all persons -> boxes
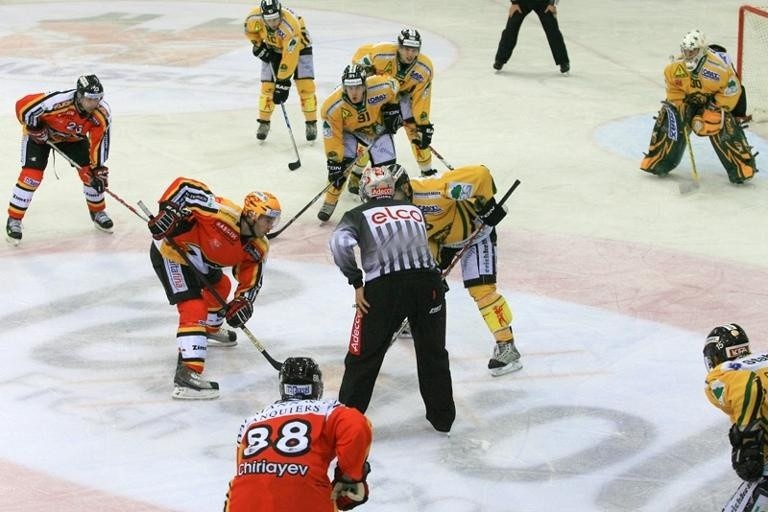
[222,353,373,512]
[243,1,318,140]
[640,30,758,184]
[348,26,435,196]
[4,74,112,240]
[149,176,279,390]
[702,322,768,512]
[376,162,521,370]
[494,0,570,73]
[315,64,398,223]
[330,165,456,432]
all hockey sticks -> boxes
[268,61,301,171]
[266,128,390,239]
[136,200,283,370]
[679,111,700,193]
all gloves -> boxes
[27,124,48,144]
[382,104,433,149]
[476,197,506,226]
[89,166,108,193]
[273,80,290,103]
[330,461,371,511]
[253,43,272,62]
[327,164,345,188]
[148,201,181,240]
[729,423,764,481]
[218,296,253,328]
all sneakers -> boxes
[7,218,22,239]
[494,61,503,69]
[395,320,410,334]
[306,120,317,140]
[257,119,270,139]
[208,328,236,342]
[91,211,113,228]
[561,62,569,72]
[489,340,520,368]
[174,368,219,390]
[348,171,362,194]
[318,202,335,221]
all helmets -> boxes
[681,29,702,50]
[703,324,749,372]
[342,64,367,86]
[243,191,281,222]
[359,164,409,200]
[279,358,323,401]
[77,75,103,99]
[259,0,281,20]
[398,28,421,48]
